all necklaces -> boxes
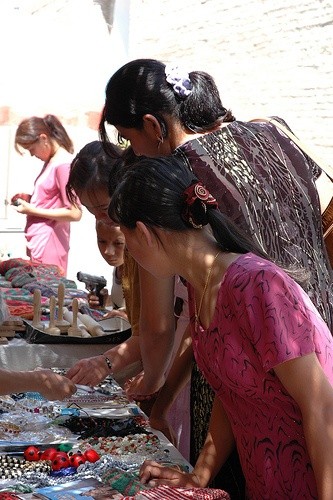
[0,368,171,489]
[194,250,222,333]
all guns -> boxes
[77,272,108,308]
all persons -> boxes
[99,58,333,500]
[109,156,333,500]
[88,218,126,318]
[66,140,191,462]
[0,370,77,401]
[11,115,82,278]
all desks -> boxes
[0,296,120,371]
[0,367,195,500]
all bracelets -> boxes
[100,351,112,372]
